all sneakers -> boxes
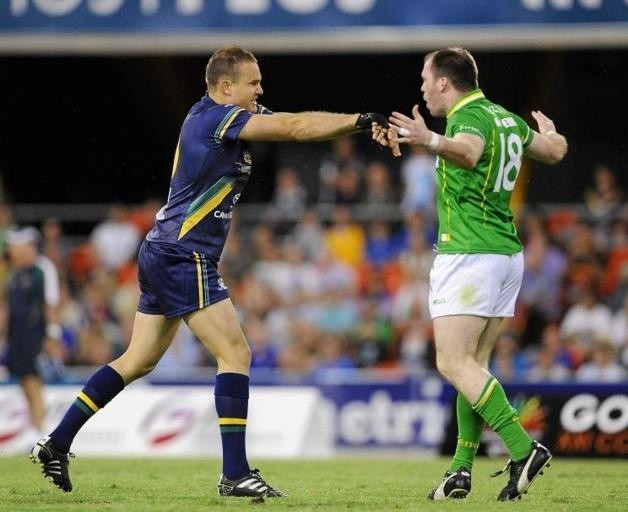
[29,435,72,492]
[217,468,289,497]
[497,439,553,501]
[426,466,471,500]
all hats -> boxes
[3,226,41,245]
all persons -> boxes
[4,225,67,435]
[385,47,568,503]
[0,118,628,383]
[27,43,403,499]
